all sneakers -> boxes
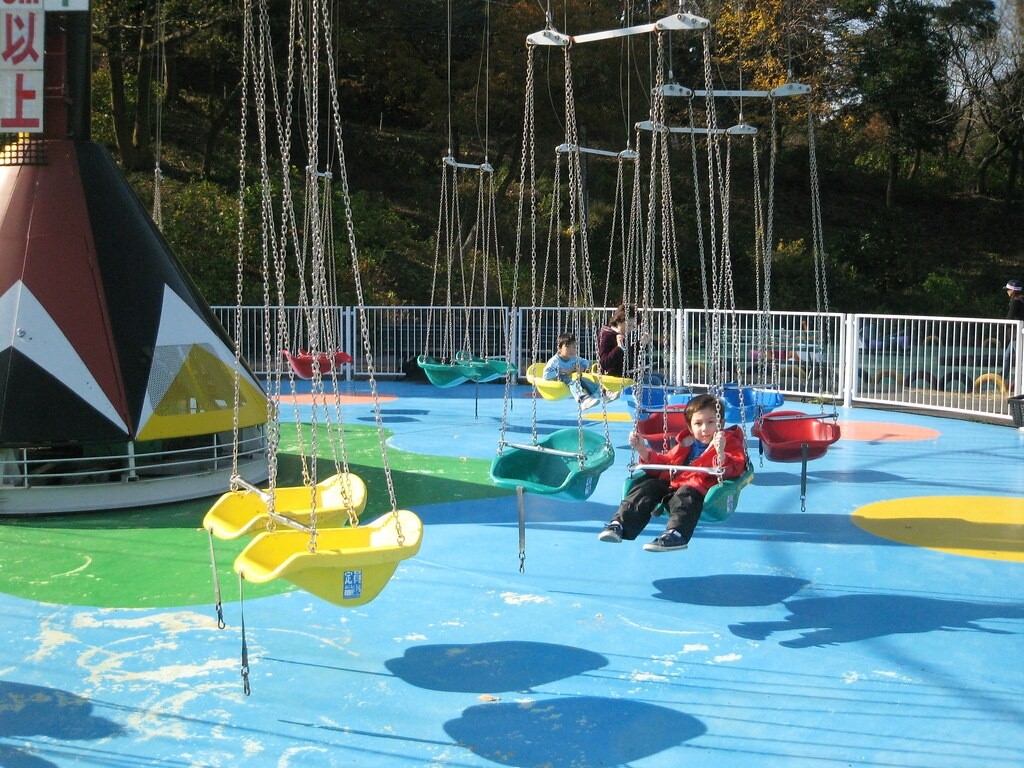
[598,521,623,545]
[644,528,690,552]
[579,395,599,411]
[602,388,620,404]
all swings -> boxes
[288,1,844,525]
[200,2,424,609]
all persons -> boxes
[1000,279,1023,380]
[599,394,746,552]
[543,333,622,410]
[597,304,674,393]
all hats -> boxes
[1003,278,1023,292]
